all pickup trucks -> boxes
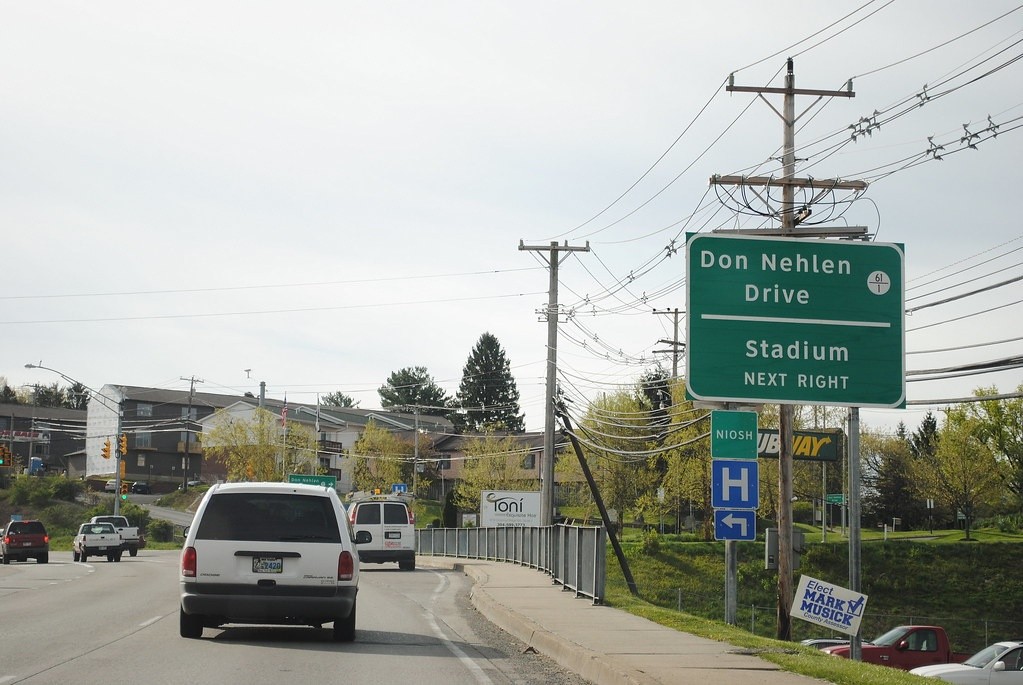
[89,515,140,557]
[819,624,974,672]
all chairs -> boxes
[921,640,931,650]
[83,529,110,533]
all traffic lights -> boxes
[121,484,128,500]
[0,446,12,466]
[118,436,127,456]
[101,440,110,459]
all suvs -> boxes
[346,499,416,572]
[71,522,123,562]
[177,481,361,643]
[0,519,49,564]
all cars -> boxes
[131,481,154,495]
[800,636,870,650]
[104,479,123,493]
[908,640,1023,685]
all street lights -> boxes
[24,363,123,516]
